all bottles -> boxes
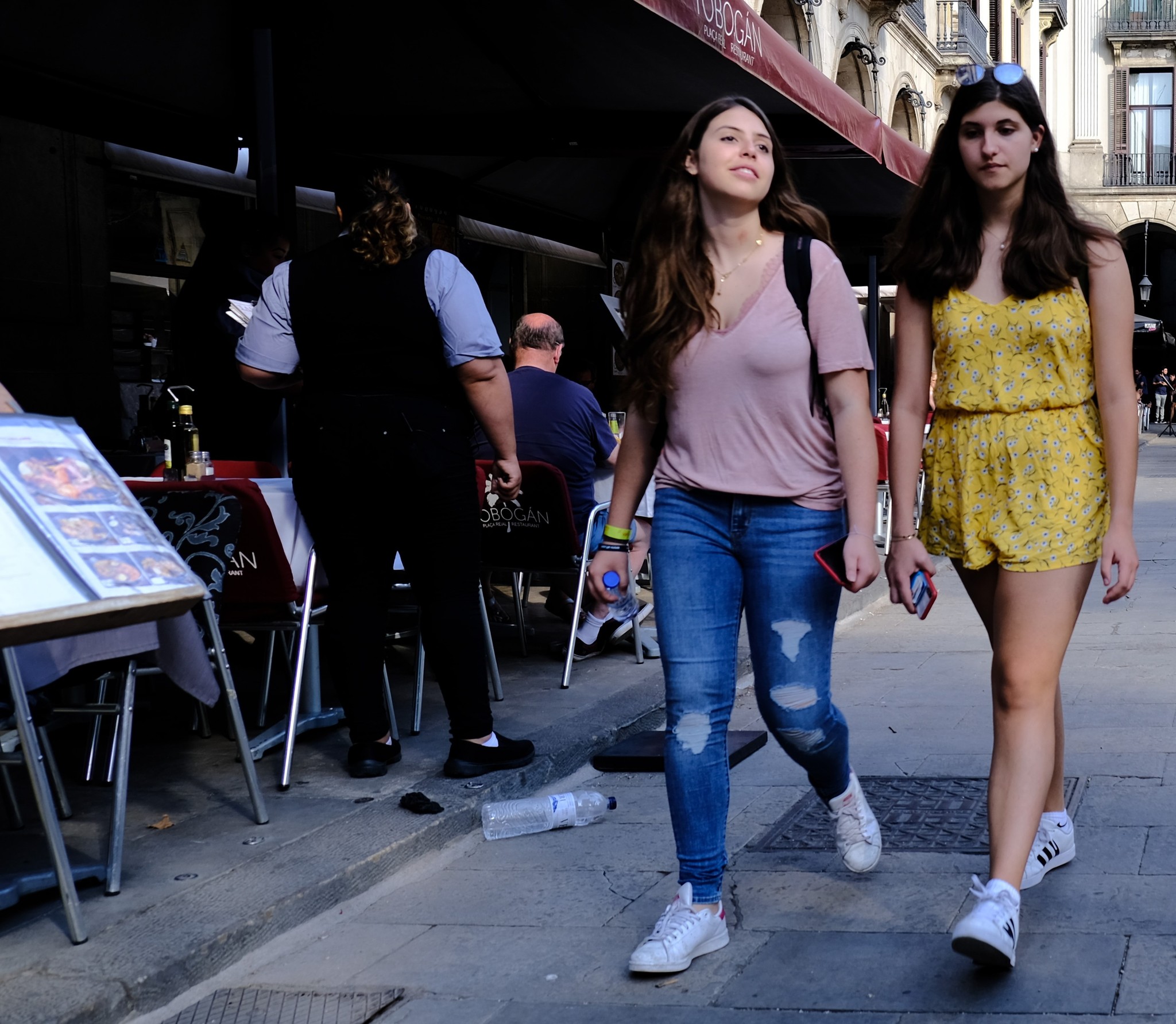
[201,451,215,481]
[481,790,616,841]
[179,404,199,461]
[186,451,205,481]
[164,400,186,482]
[602,571,640,622]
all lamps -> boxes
[1137,217,1153,307]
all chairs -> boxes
[393,543,512,738]
[147,460,401,796]
[474,458,649,689]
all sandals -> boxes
[484,591,511,624]
[544,594,587,623]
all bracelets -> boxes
[597,542,629,552]
[848,527,876,543]
[601,524,632,544]
[889,532,918,544]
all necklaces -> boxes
[984,226,1006,250]
[713,233,764,296]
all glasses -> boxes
[954,62,1029,85]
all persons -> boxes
[863,62,1145,970]
[471,304,658,661]
[1133,369,1152,431]
[1152,368,1171,424]
[162,163,539,776]
[1165,372,1176,424]
[579,96,880,974]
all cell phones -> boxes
[814,532,850,585]
[908,567,938,621]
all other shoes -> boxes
[1141,426,1147,431]
[1154,420,1166,424]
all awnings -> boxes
[0,0,983,294]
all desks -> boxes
[111,475,347,764]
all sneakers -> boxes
[950,874,1020,968]
[349,735,402,778]
[823,760,881,873]
[1019,815,1076,890]
[563,626,607,661]
[443,728,535,777]
[627,882,729,972]
